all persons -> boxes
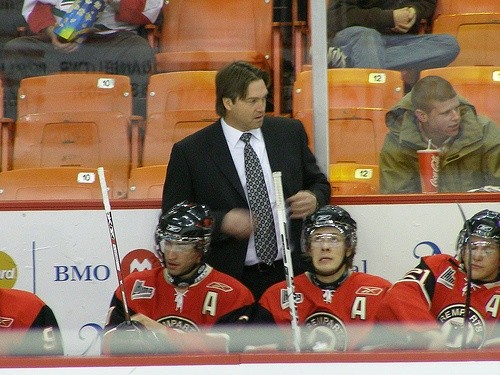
[378,75,500,195]
[0,287,64,356]
[326,0,462,92]
[0,0,165,119]
[162,60,331,302]
[102,200,500,354]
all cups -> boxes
[416,149,441,194]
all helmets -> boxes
[457,209,500,262]
[301,205,357,259]
[155,201,216,261]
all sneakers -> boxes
[308,43,351,69]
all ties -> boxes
[240,133,278,266]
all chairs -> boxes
[0,0,500,200]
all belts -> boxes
[244,259,283,273]
